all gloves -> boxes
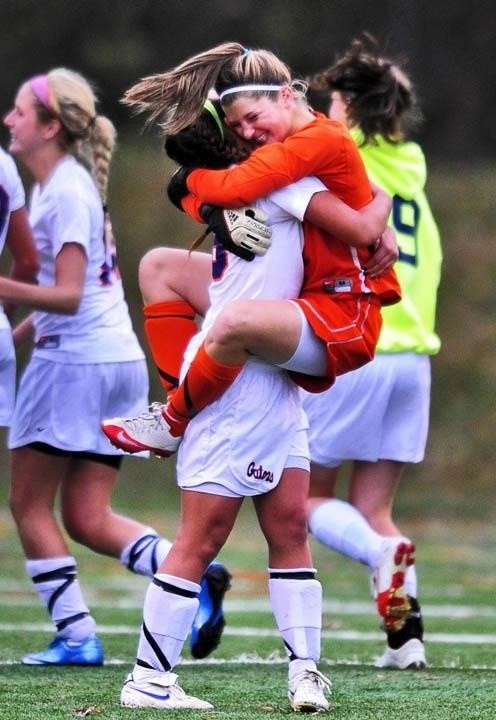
[207,207,273,262]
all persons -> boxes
[293,28,447,673]
[1,147,42,426]
[0,65,237,667]
[118,86,403,716]
[98,40,406,458]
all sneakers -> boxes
[22,637,103,666]
[370,536,416,632]
[374,638,427,670]
[120,672,214,709]
[101,403,183,460]
[287,658,333,713]
[190,562,232,659]
